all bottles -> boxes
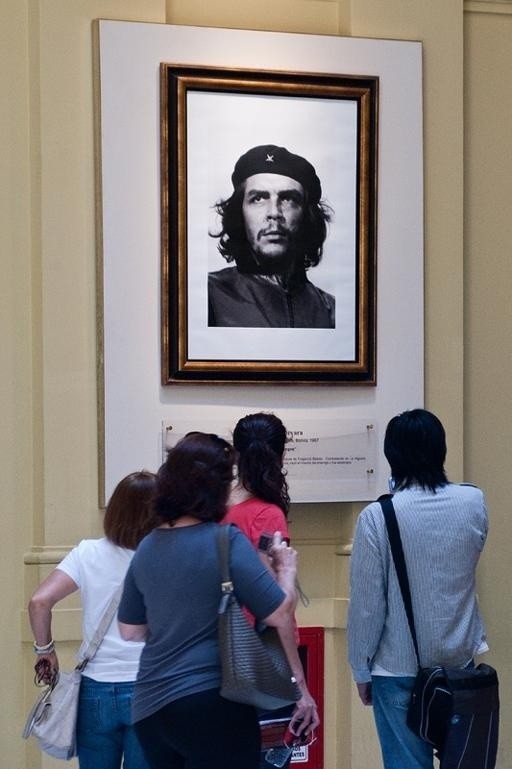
[263,706,303,768]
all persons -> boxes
[342,407,490,768]
[213,412,319,769]
[206,141,336,329]
[26,471,163,769]
[116,430,299,769]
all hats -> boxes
[231,145,321,191]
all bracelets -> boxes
[33,639,56,655]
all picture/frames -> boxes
[160,63,377,389]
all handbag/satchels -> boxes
[23,668,82,761]
[220,593,303,711]
[406,665,498,751]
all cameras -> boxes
[388,477,396,493]
[257,533,274,554]
